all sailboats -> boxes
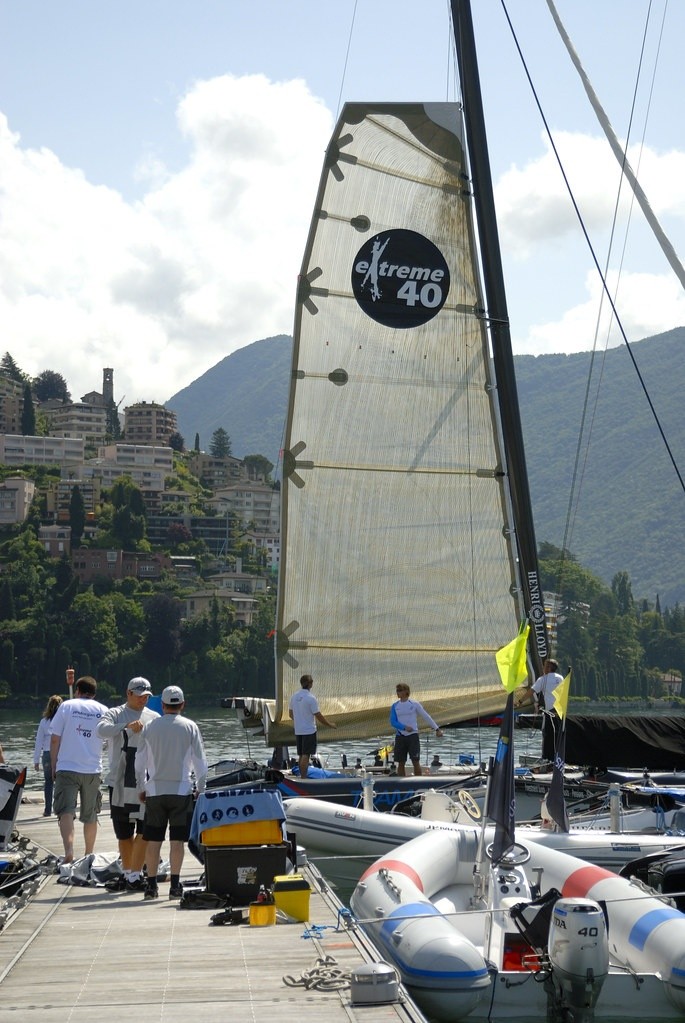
[185,0,685,908]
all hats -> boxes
[161,685,184,704]
[128,676,153,697]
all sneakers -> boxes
[145,885,159,899]
[106,874,128,892]
[125,875,147,893]
[169,883,184,899]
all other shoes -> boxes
[43,812,51,816]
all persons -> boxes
[390,684,443,776]
[35,695,77,819]
[0,745,5,763]
[514,659,563,773]
[289,675,337,778]
[95,677,159,893]
[50,677,108,871]
[135,686,208,899]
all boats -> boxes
[347,827,684,1023]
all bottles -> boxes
[257,884,275,904]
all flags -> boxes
[496,623,530,694]
[552,673,571,719]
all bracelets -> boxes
[436,728,440,731]
[519,700,523,704]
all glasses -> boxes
[131,685,151,691]
[397,689,403,691]
